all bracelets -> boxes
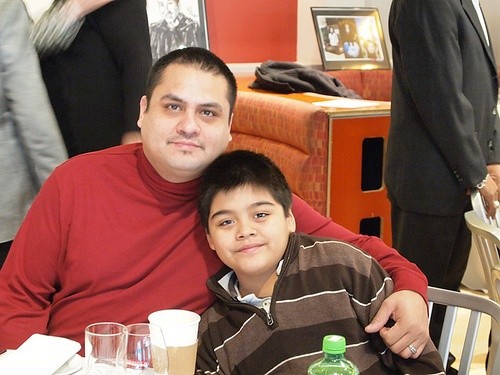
[474,173,489,188]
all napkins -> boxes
[4,333,81,375]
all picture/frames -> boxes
[144,0,209,58]
[312,7,390,72]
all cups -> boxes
[123,322,154,374]
[84,322,128,374]
[148,309,201,347]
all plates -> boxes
[52,354,84,375]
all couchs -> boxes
[229,70,392,246]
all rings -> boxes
[493,200,499,209]
[409,345,416,354]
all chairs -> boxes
[465,191,500,305]
[425,286,500,374]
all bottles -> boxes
[307,334,360,375]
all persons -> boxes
[388,0,500,366]
[196,150,447,375]
[0,47,429,370]
[30,0,153,159]
[0,0,67,267]
[328,26,376,54]
